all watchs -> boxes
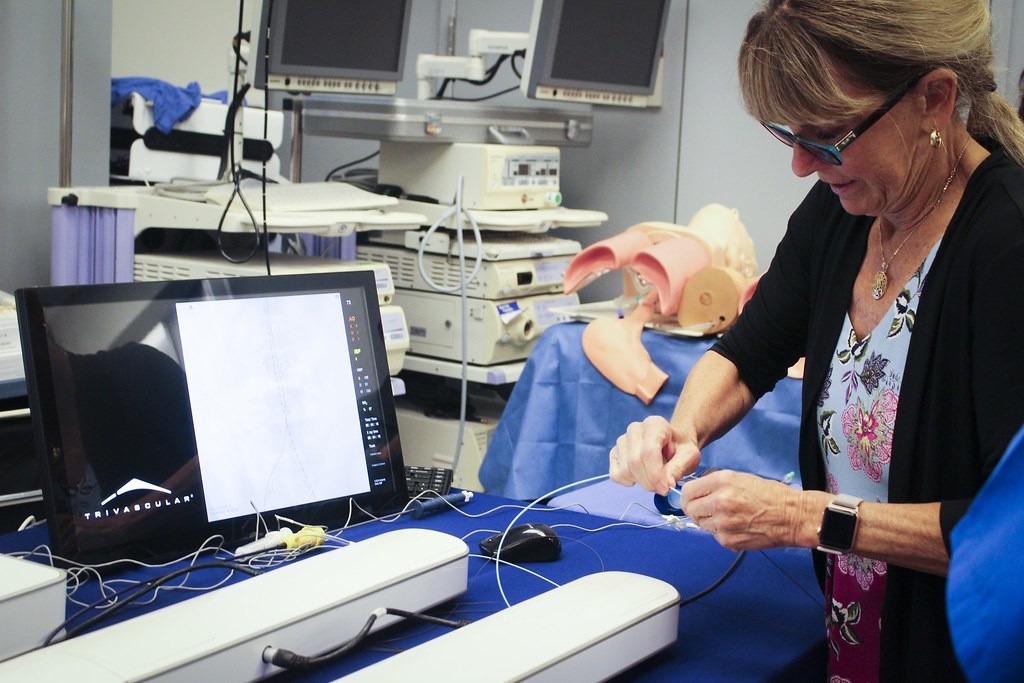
[817,493,862,558]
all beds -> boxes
[476,318,806,510]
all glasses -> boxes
[760,67,938,165]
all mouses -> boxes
[479,523,562,564]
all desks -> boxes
[0,486,828,683]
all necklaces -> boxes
[870,136,973,300]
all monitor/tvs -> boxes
[12,269,406,585]
[518,0,671,110]
[268,0,413,81]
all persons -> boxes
[607,1,1024,683]
[48,325,197,543]
